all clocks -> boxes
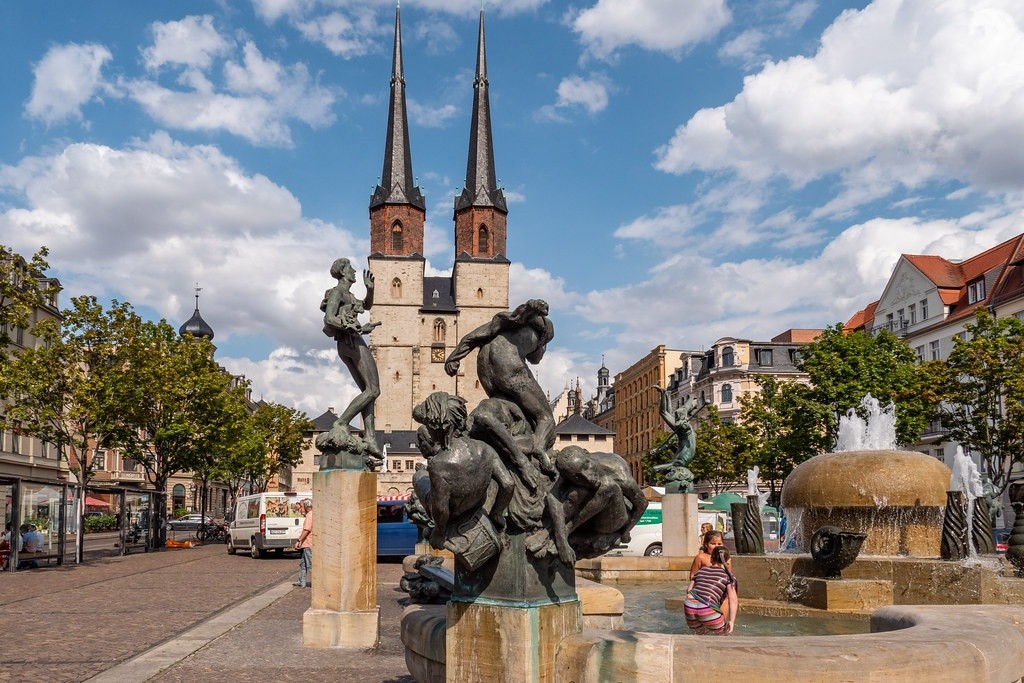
[431,343,446,363]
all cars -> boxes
[602,501,662,558]
[376,501,419,557]
[85,510,103,518]
[166,513,215,531]
[992,526,1012,553]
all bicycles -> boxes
[197,521,231,541]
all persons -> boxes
[325,257,386,459]
[0,521,44,570]
[698,523,714,548]
[689,529,733,620]
[411,299,650,571]
[682,546,739,636]
[292,499,313,588]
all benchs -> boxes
[0,547,48,572]
[114,542,149,556]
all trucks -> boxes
[227,492,312,560]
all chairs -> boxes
[380,509,390,523]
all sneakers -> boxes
[291,581,305,587]
